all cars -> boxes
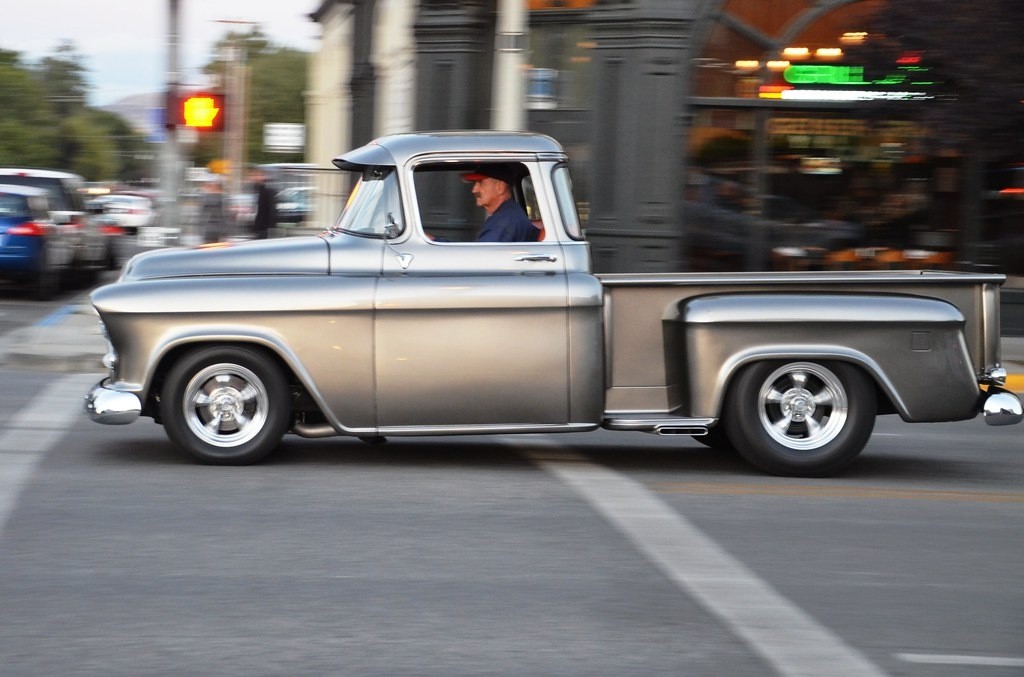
[0,169,317,302]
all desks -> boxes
[773,247,956,270]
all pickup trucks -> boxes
[81,129,1024,480]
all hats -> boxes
[456,164,521,185]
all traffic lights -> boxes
[182,93,225,133]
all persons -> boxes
[199,181,231,244]
[240,167,276,239]
[424,164,532,242]
